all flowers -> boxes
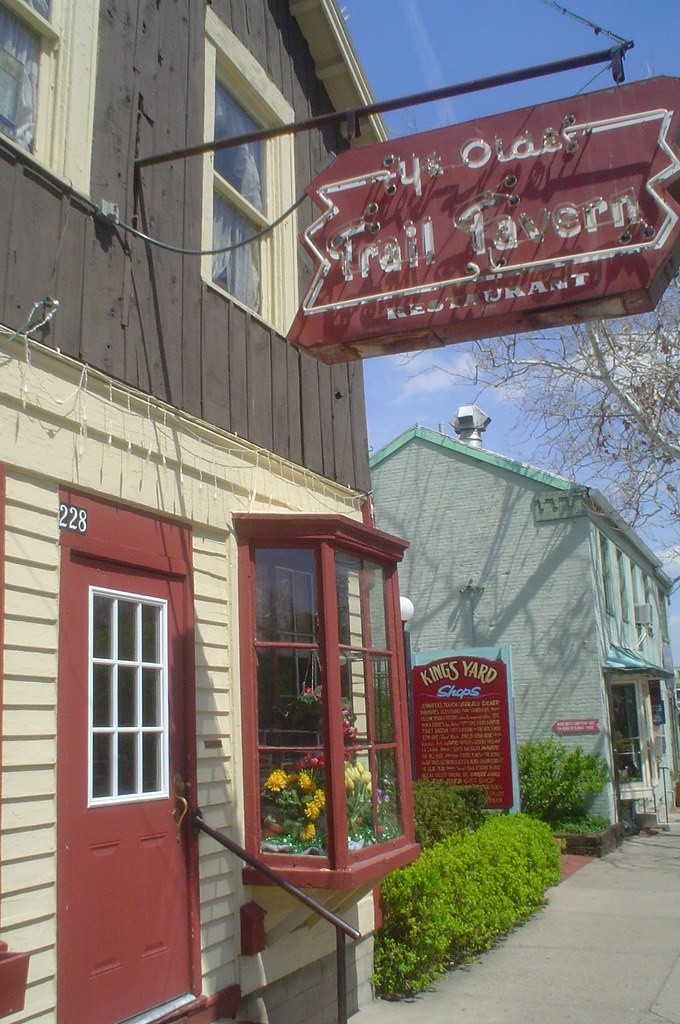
[274,687,357,745]
[261,749,391,842]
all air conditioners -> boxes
[634,603,653,624]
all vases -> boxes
[284,818,304,834]
[261,798,291,825]
